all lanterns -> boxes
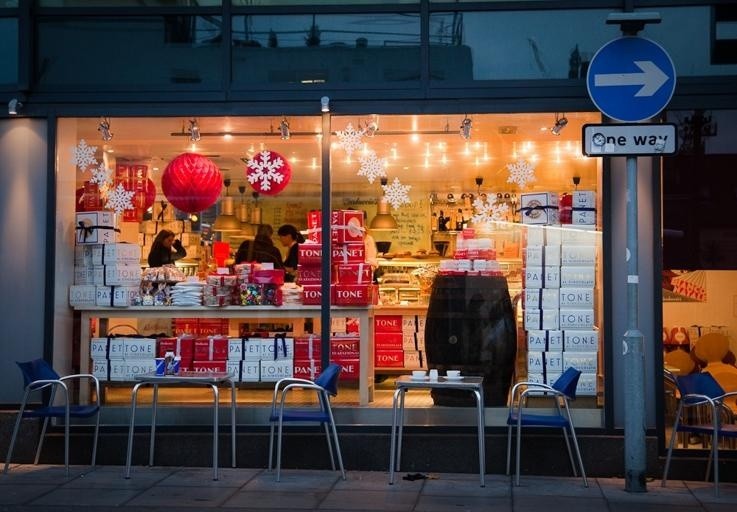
[162,153,223,214]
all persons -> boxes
[278,225,305,282]
[235,224,283,269]
[148,229,186,267]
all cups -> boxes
[447,370,461,377]
[155,358,166,377]
[429,369,439,380]
[174,357,183,375]
[412,371,427,377]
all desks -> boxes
[370,306,436,395]
[122,369,239,480]
[388,374,492,488]
[75,305,377,408]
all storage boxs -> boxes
[90,334,357,390]
[293,210,381,305]
[76,215,144,308]
[522,193,599,397]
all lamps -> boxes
[552,111,568,137]
[188,116,201,142]
[216,178,266,241]
[368,176,399,233]
[98,117,114,142]
[461,113,474,139]
[278,116,290,140]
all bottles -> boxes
[164,350,176,375]
[432,211,450,230]
[456,209,463,230]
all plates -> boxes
[443,377,465,381]
[410,377,430,381]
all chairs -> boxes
[267,362,347,484]
[660,371,736,498]
[504,365,590,491]
[4,356,104,478]
